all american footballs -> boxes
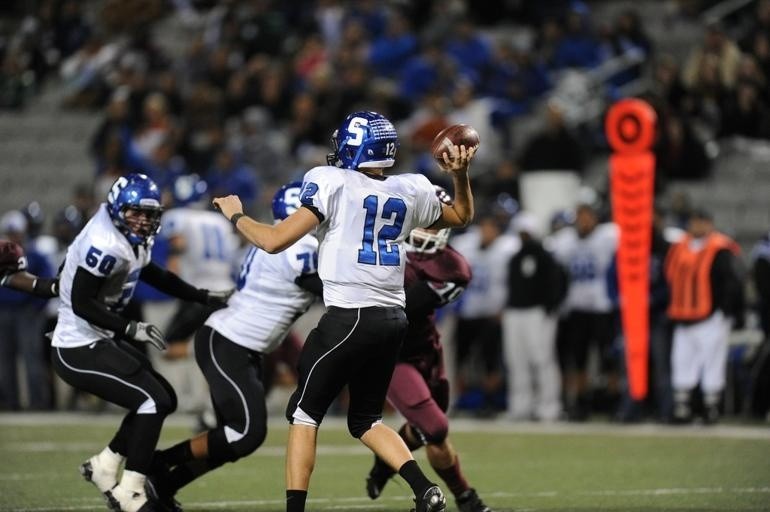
[431,123,480,164]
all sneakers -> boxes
[78,455,181,511]
[367,454,395,499]
[411,483,446,511]
[455,488,491,512]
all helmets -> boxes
[404,184,454,254]
[326,110,400,170]
[272,181,302,221]
[107,174,165,247]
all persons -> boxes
[363,226,491,512]
[213,109,479,512]
[1,0,770,426]
[44,172,231,512]
[144,178,325,512]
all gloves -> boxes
[208,286,236,310]
[127,320,168,351]
